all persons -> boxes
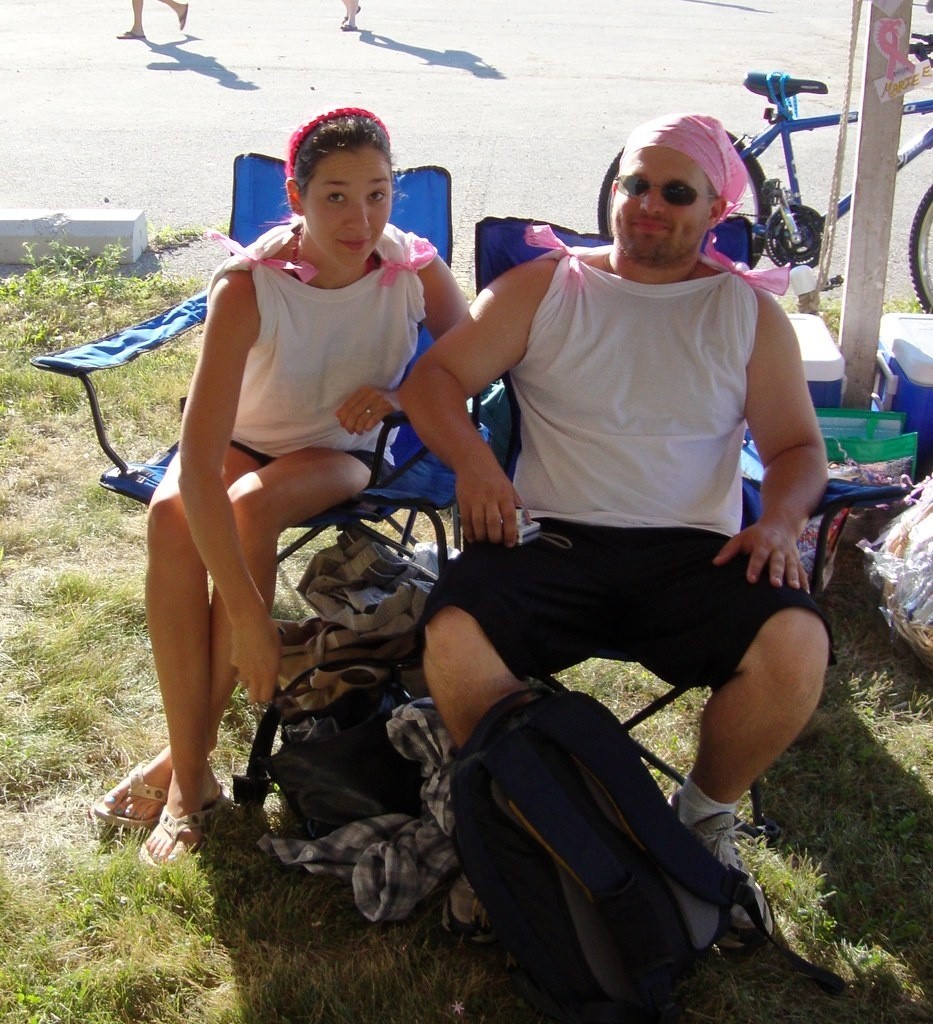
[117,0,189,39]
[340,0,362,30]
[93,108,472,870]
[400,114,833,949]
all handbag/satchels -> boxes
[232,659,427,842]
[816,408,917,502]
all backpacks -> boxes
[448,687,847,1024]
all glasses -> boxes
[614,176,717,206]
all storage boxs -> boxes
[785,313,847,409]
[869,312,932,464]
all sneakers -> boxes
[668,791,774,952]
[442,871,495,945]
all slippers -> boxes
[138,785,231,872]
[92,760,170,829]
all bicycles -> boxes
[594,34,933,314]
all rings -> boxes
[365,408,372,416]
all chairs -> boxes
[30,150,454,565]
[363,214,910,841]
